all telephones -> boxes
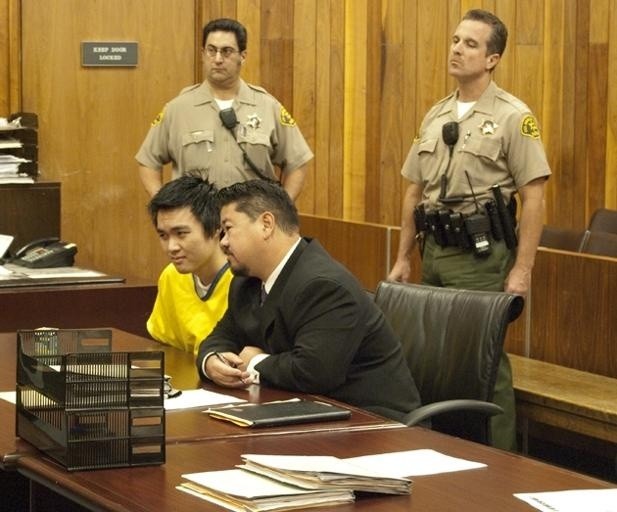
[12,237,78,269]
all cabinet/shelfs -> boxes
[0,112,40,176]
[0,182,62,253]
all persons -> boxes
[387,10,552,453]
[196,180,432,429]
[135,19,314,204]
[146,175,234,356]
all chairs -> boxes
[536,205,617,259]
[363,279,526,448]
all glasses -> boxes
[202,47,241,56]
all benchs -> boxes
[499,353,617,456]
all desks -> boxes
[0,322,407,473]
[16,422,617,512]
[0,267,159,337]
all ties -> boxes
[257,284,268,309]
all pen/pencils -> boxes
[215,351,247,385]
